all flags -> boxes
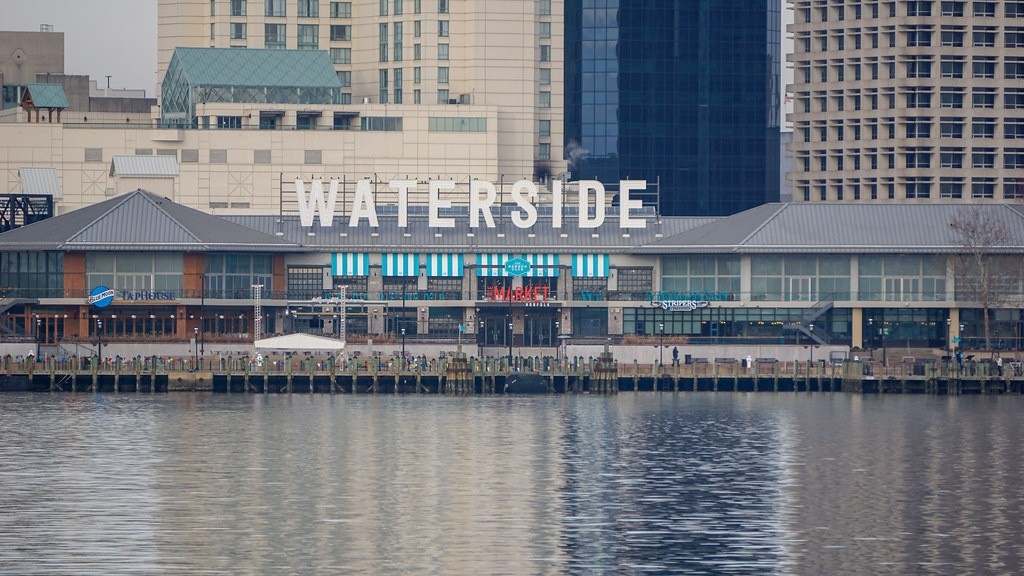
[953,337,960,343]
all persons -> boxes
[422,353,428,367]
[995,356,1002,376]
[957,347,965,373]
[671,347,679,367]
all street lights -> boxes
[947,318,951,371]
[659,323,663,367]
[869,318,874,361]
[960,324,964,365]
[809,324,814,367]
[509,323,513,366]
[401,328,405,358]
[98,321,103,366]
[37,319,41,362]
[480,321,485,361]
[194,328,200,371]
[555,321,559,362]
